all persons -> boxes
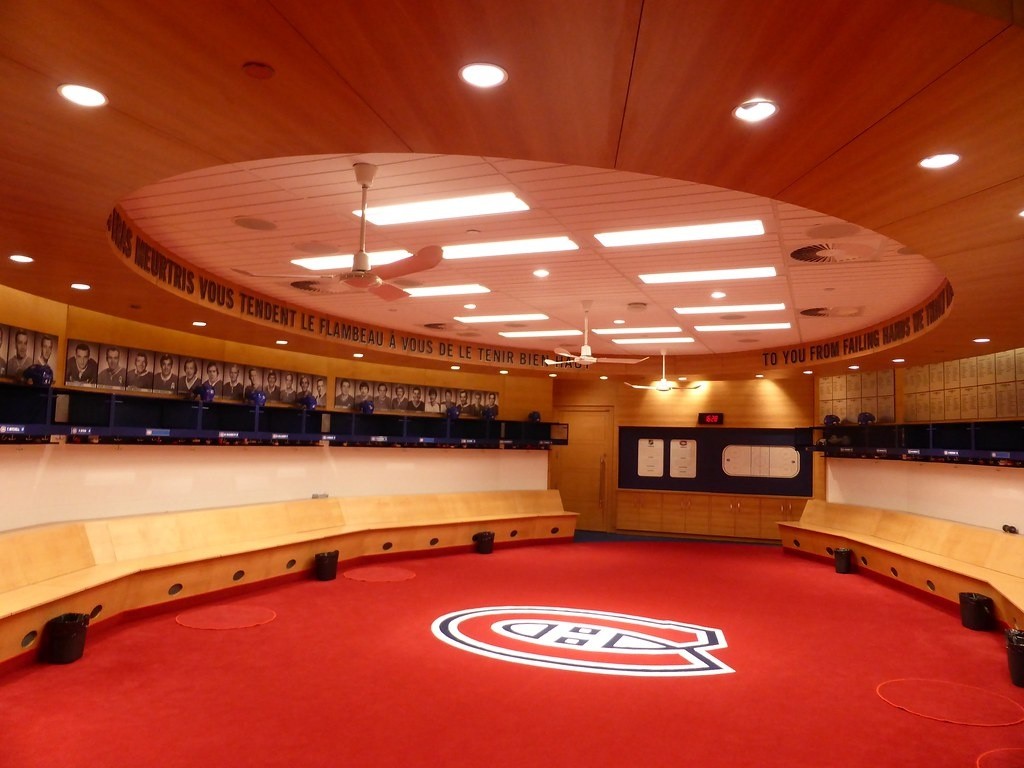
[0,330,501,430]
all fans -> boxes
[542,309,651,367]
[238,161,442,304]
[624,346,701,391]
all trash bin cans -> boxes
[958,592,992,630]
[834,548,852,573]
[314,552,338,581]
[475,531,492,554]
[43,612,87,664]
[1007,629,1024,687]
